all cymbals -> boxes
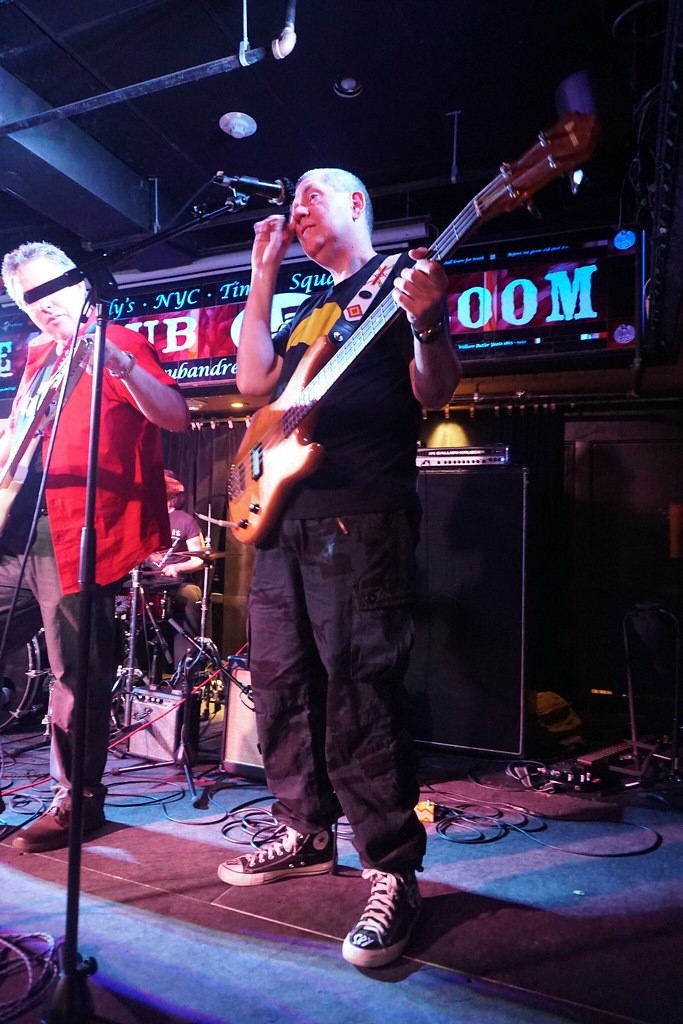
[170,547,227,560]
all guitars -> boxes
[226,111,601,543]
[0,320,98,549]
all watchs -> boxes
[410,308,445,345]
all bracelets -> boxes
[109,350,136,379]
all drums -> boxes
[0,626,52,735]
[112,574,181,623]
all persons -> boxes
[218,168,463,966]
[114,469,208,695]
[0,240,191,853]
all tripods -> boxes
[112,503,221,806]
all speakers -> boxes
[124,685,202,764]
[561,421,683,699]
[216,661,266,780]
[402,467,530,756]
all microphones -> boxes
[213,173,296,208]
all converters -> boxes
[413,800,435,823]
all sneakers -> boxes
[217,824,337,886]
[343,868,423,967]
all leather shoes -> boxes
[12,802,106,852]
